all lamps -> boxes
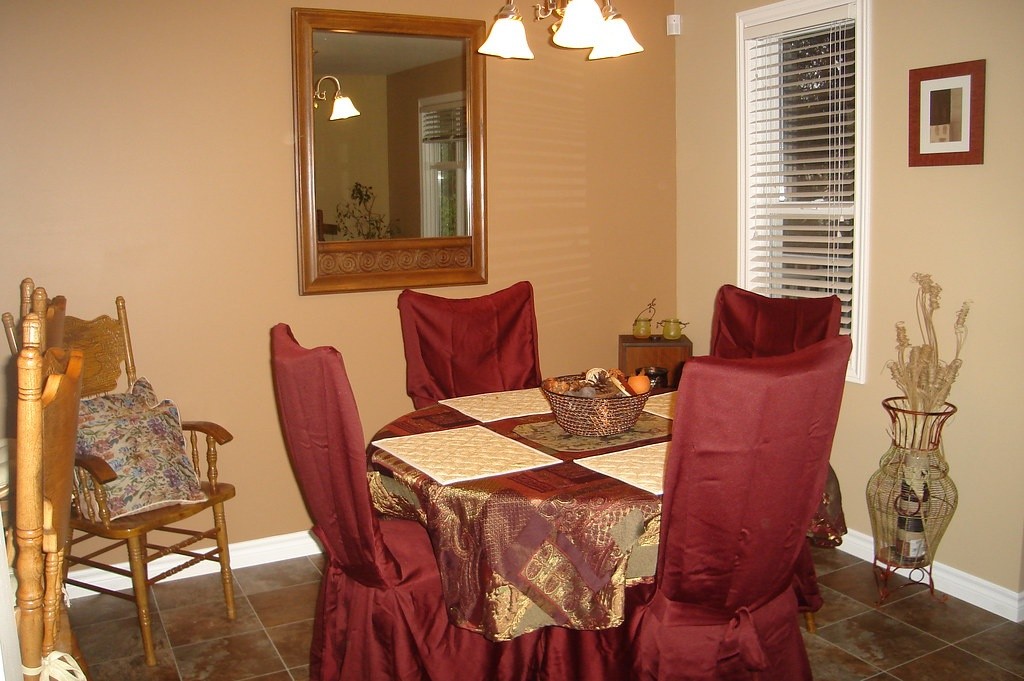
[478,1,534,60]
[534,0,646,62]
[313,75,361,121]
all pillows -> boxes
[67,373,209,521]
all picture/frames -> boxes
[909,59,986,167]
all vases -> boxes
[865,396,959,568]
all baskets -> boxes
[541,374,652,437]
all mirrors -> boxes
[290,7,489,296]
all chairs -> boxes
[0,278,94,681]
[710,283,841,359]
[269,322,853,681]
[397,281,542,410]
[51,295,236,668]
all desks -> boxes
[619,333,693,384]
[365,385,679,646]
